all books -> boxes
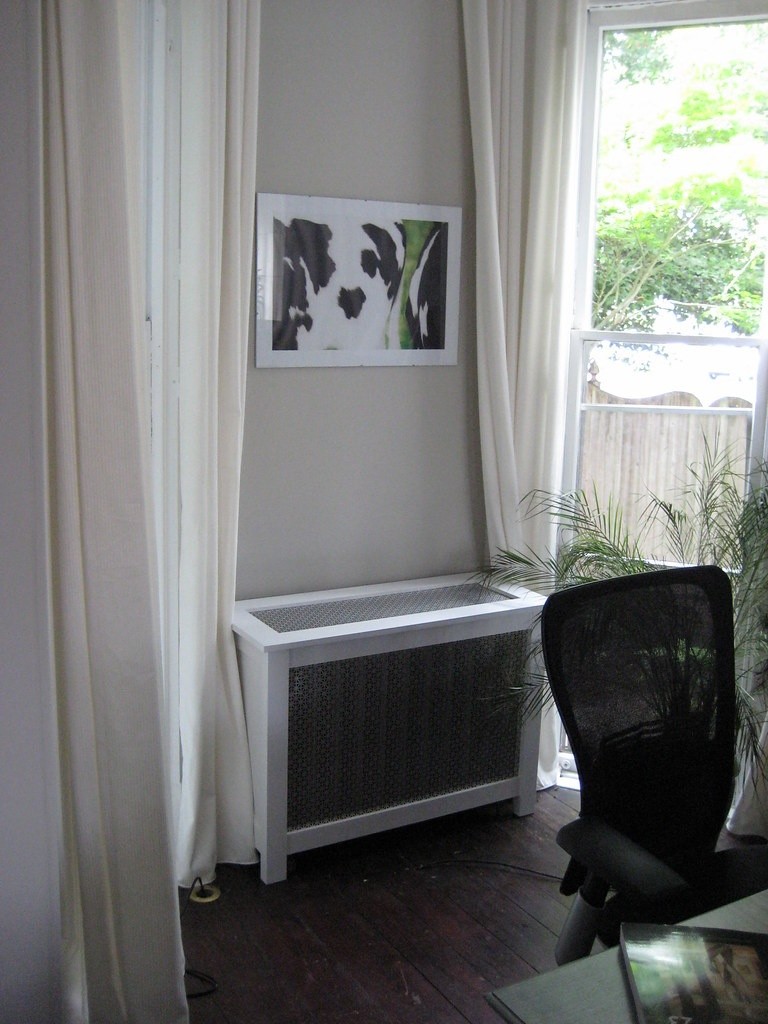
[620,921,768,1024]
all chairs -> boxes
[540,565,768,965]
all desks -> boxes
[483,889,768,1024]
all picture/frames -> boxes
[256,192,462,368]
